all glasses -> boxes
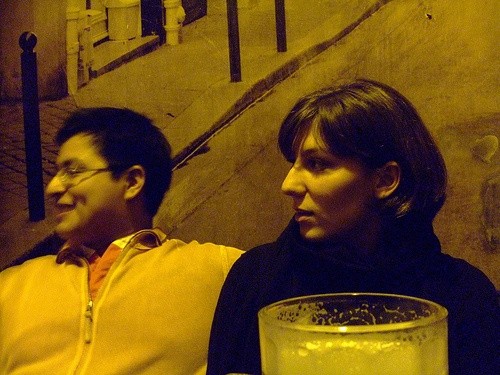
[55,166,114,183]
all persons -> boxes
[1,103,248,375]
[198,77,499,373]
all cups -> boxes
[257,293,450,375]
